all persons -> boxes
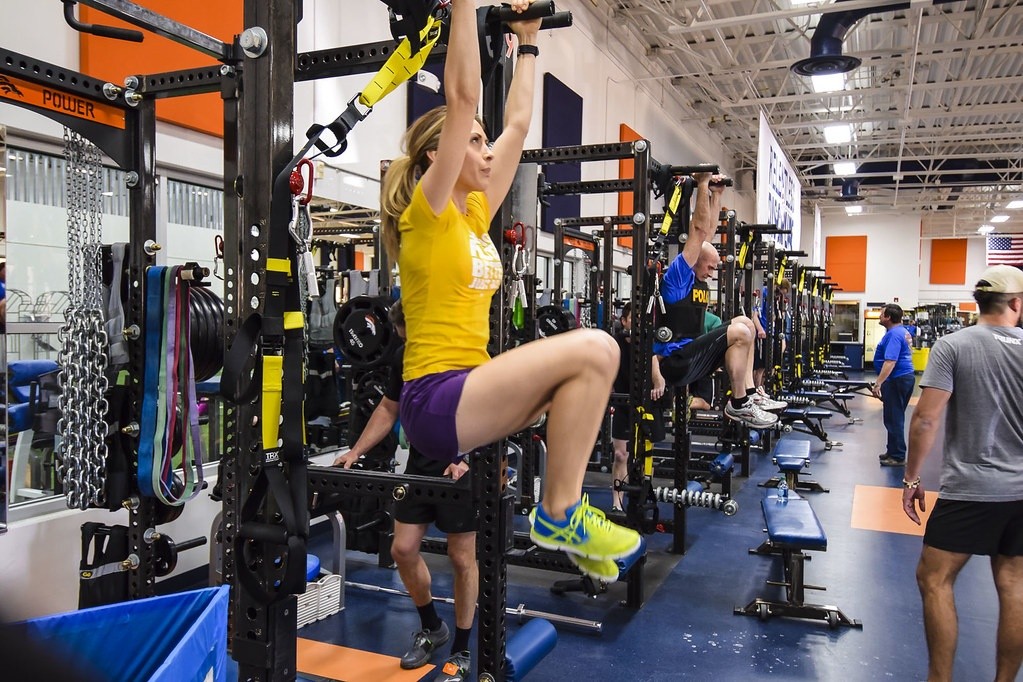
[610,302,665,512]
[872,304,915,465]
[902,265,1023,682]
[333,302,479,682]
[654,163,788,429]
[753,277,791,385]
[906,320,917,346]
[380,0,643,586]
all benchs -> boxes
[733,487,863,631]
[779,365,882,450]
[756,439,831,498]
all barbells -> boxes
[189,284,227,382]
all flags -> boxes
[985,236,1023,266]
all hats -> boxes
[974,265,1023,293]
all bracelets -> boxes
[902,476,921,489]
[516,45,540,58]
[874,383,880,388]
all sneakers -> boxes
[528,506,619,582]
[725,396,778,428]
[879,452,891,458]
[401,622,450,668]
[881,457,905,466]
[435,650,471,682]
[530,492,642,561]
[750,386,788,411]
